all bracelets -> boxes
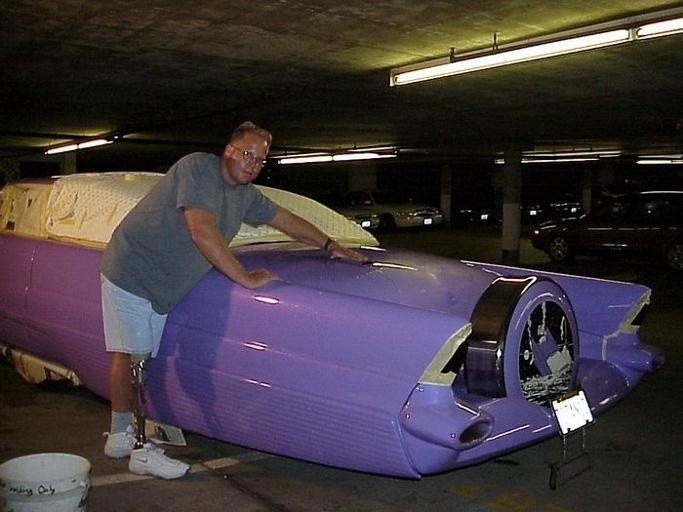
[325,238,332,250]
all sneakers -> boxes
[129,441,192,480]
[102,425,165,458]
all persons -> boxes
[98,120,367,481]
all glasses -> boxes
[238,150,270,169]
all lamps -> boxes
[385,2,681,92]
[43,133,121,155]
[278,145,403,165]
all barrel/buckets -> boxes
[0,453,94,512]
